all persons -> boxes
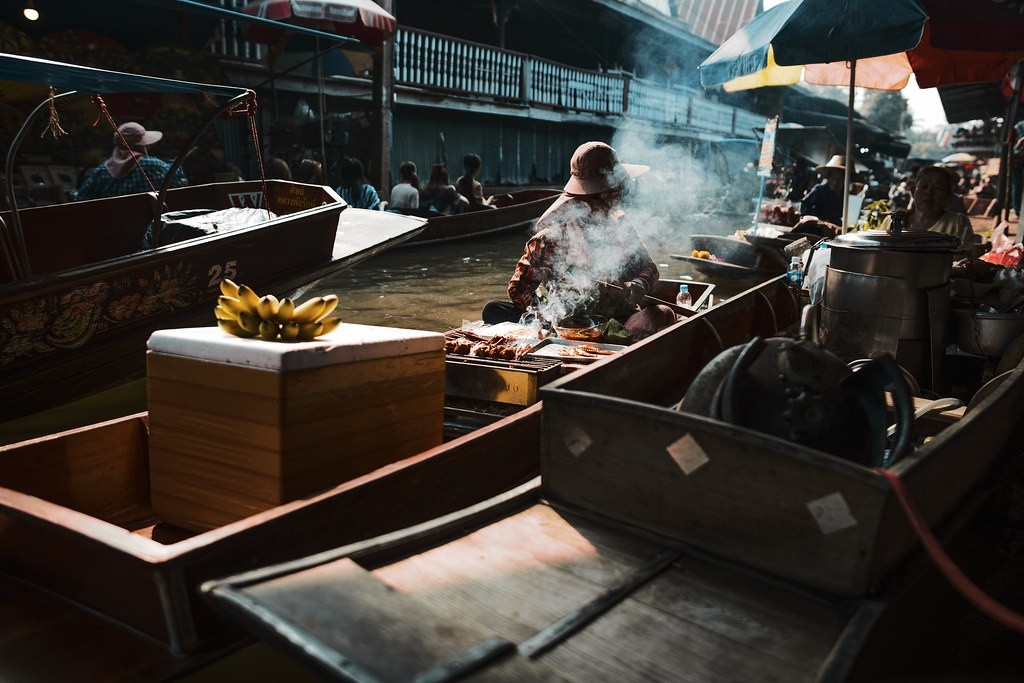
[454,153,497,212]
[977,177,994,198]
[481,141,675,344]
[267,159,291,180]
[421,164,470,214]
[336,157,389,211]
[1012,120,1024,219]
[880,165,973,249]
[298,158,324,185]
[77,122,187,200]
[800,155,855,226]
[390,161,421,209]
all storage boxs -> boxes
[144,328,449,533]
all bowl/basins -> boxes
[953,308,1024,356]
[554,326,607,342]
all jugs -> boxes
[675,336,914,471]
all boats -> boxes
[0,50,430,425]
[390,187,568,249]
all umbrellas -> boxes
[236,0,397,160]
[698,0,1024,235]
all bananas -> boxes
[214,278,342,340]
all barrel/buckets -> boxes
[822,211,962,399]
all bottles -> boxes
[787,257,802,290]
[676,285,692,321]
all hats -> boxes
[113,122,163,146]
[816,155,857,179]
[563,142,649,195]
